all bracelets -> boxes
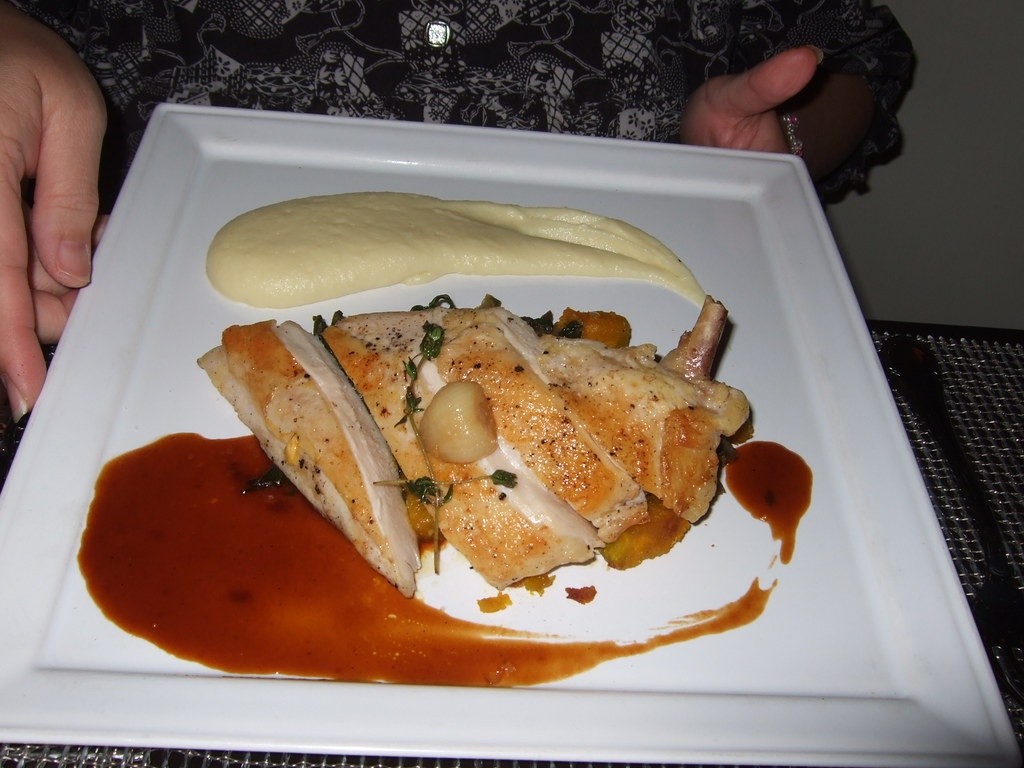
[777,106,804,155]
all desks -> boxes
[0,318,1024,768]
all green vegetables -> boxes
[242,294,517,573]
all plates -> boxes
[1,104,1020,767]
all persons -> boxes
[0,0,918,423]
